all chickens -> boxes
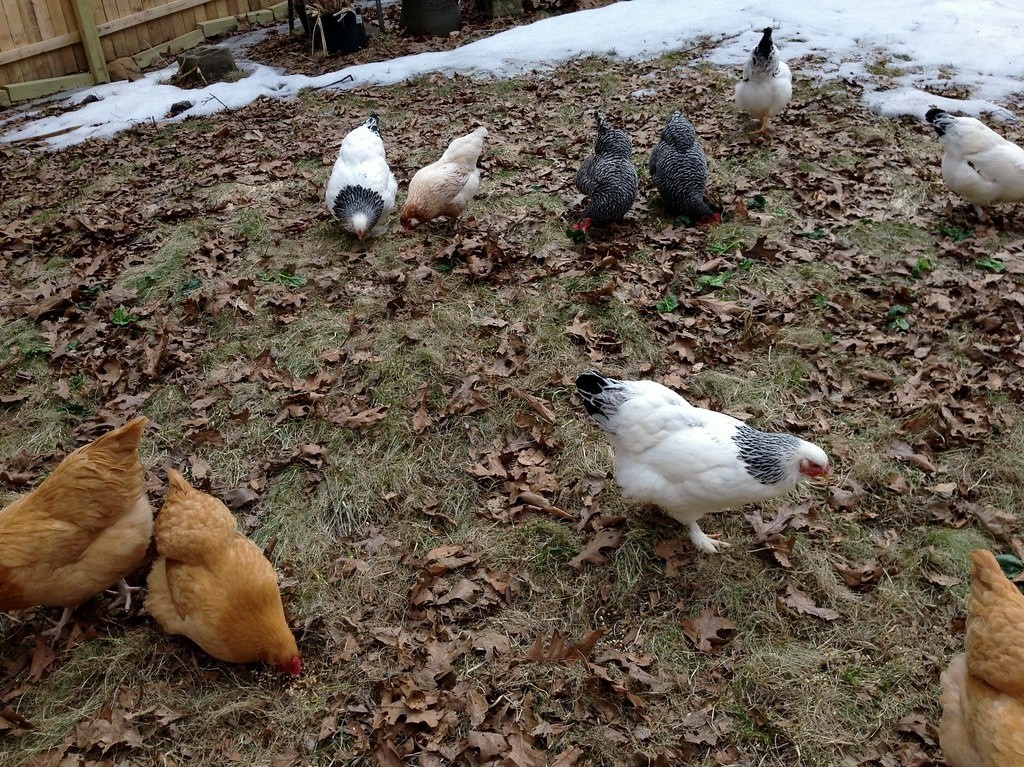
[936,548,1023,767]
[575,370,832,555]
[735,27,793,134]
[650,114,724,227]
[324,114,397,241]
[926,109,1024,222]
[400,127,488,231]
[572,111,638,234]
[0,414,302,676]
